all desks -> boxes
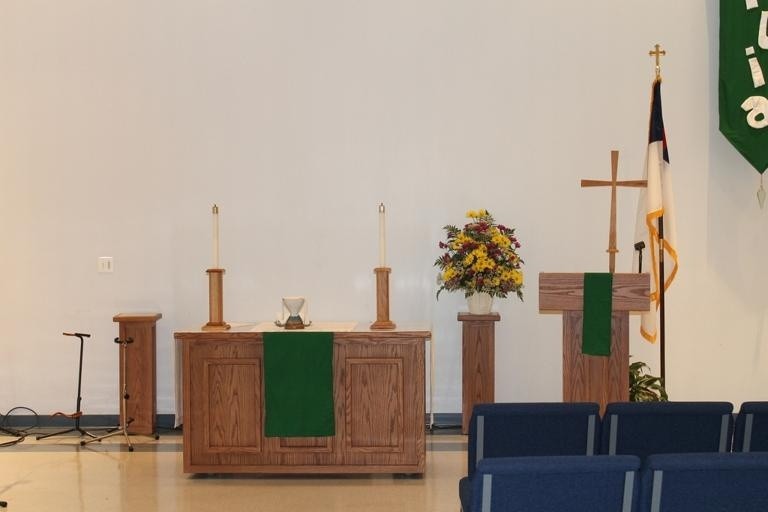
[170,321,433,482]
[109,310,163,440]
[455,311,501,437]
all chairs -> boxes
[728,402,767,454]
[640,452,768,510]
[599,401,734,459]
[459,455,643,511]
[467,402,602,477]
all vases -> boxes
[462,288,496,318]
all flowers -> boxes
[429,207,528,307]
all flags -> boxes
[718,0,768,210]
[631,76,679,343]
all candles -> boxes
[208,201,223,268]
[373,198,389,270]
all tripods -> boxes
[80,337,159,451]
[37,332,102,442]
